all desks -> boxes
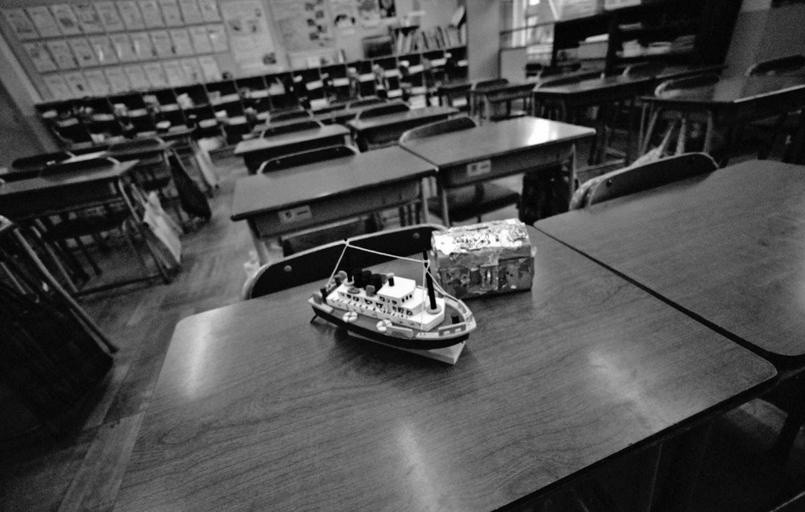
[2,217,116,470]
[110,221,778,511]
[534,159,805,377]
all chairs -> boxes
[1,55,805,306]
